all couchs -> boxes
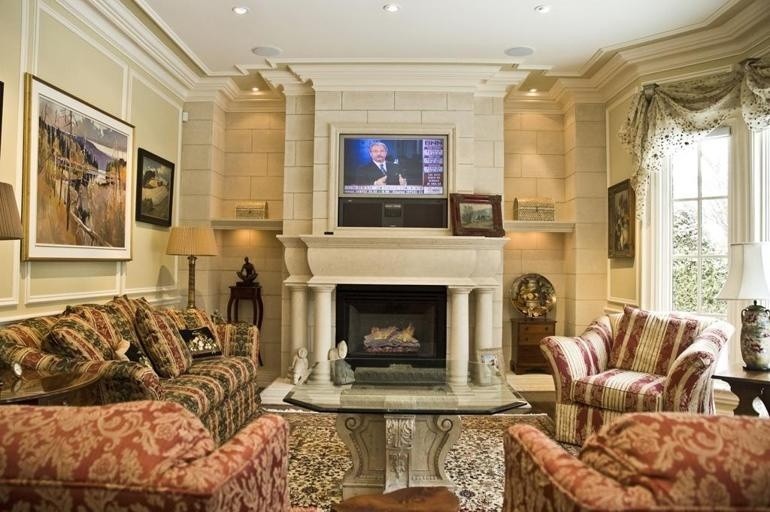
[538,311,736,448]
[0,313,261,446]
[0,400,290,512]
[502,412,770,512]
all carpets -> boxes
[264,408,584,511]
[513,391,740,423]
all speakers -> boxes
[338,198,448,227]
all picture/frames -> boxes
[19,73,136,262]
[475,347,506,386]
[608,179,636,258]
[450,193,505,237]
[135,147,175,226]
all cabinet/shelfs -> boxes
[510,318,557,375]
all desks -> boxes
[227,286,264,366]
[0,369,103,405]
[711,364,770,417]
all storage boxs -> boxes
[236,200,268,219]
[513,196,554,221]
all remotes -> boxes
[323,231,334,235]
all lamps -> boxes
[165,226,218,308]
[715,242,770,371]
[0,182,25,239]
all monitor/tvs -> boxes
[338,134,448,198]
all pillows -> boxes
[41,295,222,378]
[607,305,698,377]
